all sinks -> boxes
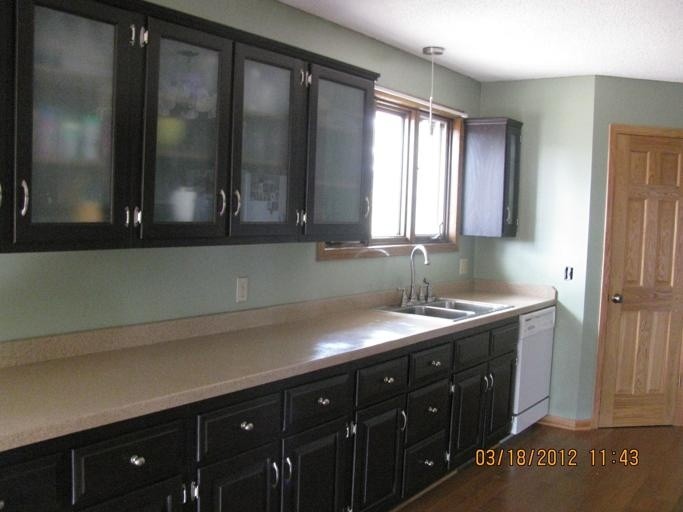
[374,299,473,321]
[426,297,515,313]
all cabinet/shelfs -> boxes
[0,0,136,253]
[349,332,455,512]
[453,313,519,471]
[1,405,195,512]
[459,116,524,237]
[136,0,311,249]
[308,50,380,245]
[192,361,351,512]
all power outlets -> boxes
[235,276,248,303]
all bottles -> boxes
[153,184,215,222]
[34,105,101,168]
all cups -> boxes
[69,198,104,223]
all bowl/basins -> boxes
[156,117,191,152]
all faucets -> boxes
[407,243,431,302]
